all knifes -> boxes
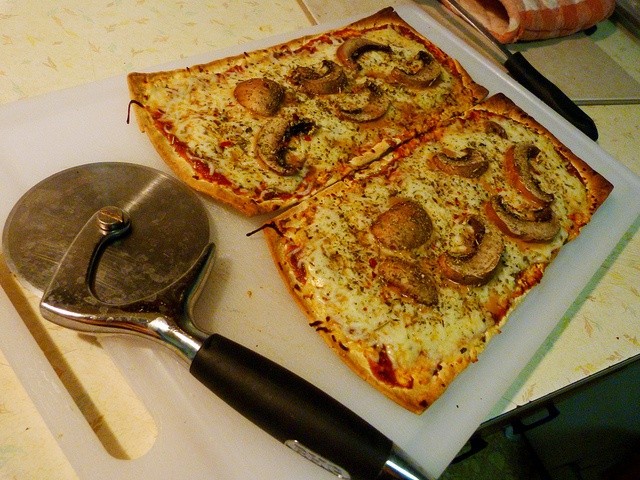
[448,0,599,142]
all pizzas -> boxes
[244,90,615,414]
[124,4,490,222]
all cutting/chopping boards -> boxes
[0,0,640,480]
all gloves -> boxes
[455,1,617,44]
